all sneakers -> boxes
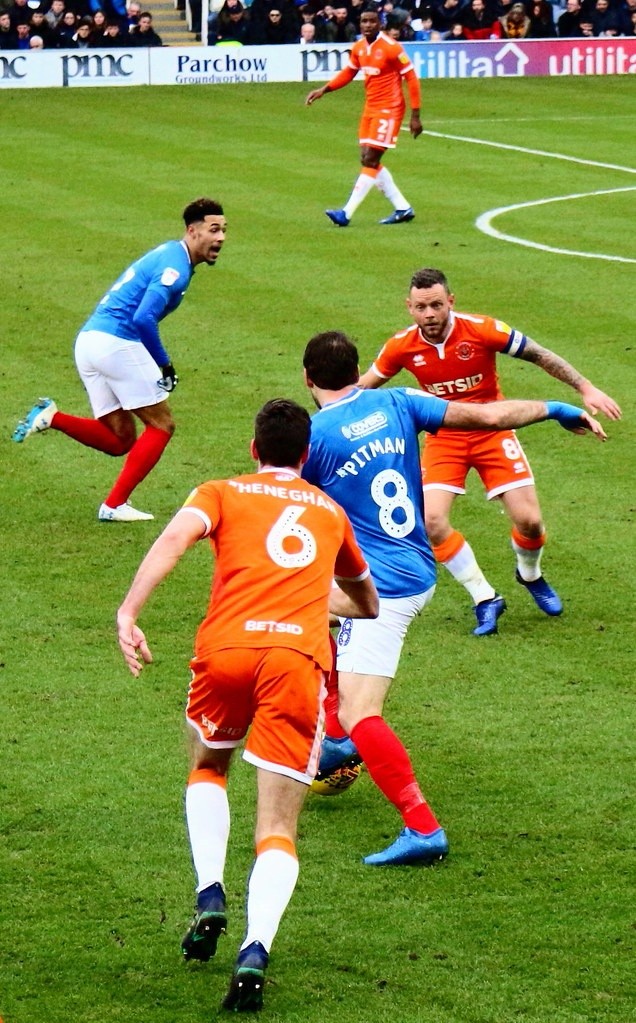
[326,208,350,227]
[473,591,508,635]
[316,737,358,770]
[13,397,59,442]
[180,882,228,960]
[223,939,271,1013]
[516,568,564,616]
[381,207,415,224]
[99,499,156,523]
[364,827,449,866]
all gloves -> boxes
[156,367,179,392]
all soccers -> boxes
[309,763,361,796]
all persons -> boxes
[116,397,378,1012]
[0,0,163,49]
[13,199,228,522]
[208,0,636,46]
[302,332,608,866]
[357,268,622,636]
[306,8,423,227]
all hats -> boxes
[230,5,242,14]
